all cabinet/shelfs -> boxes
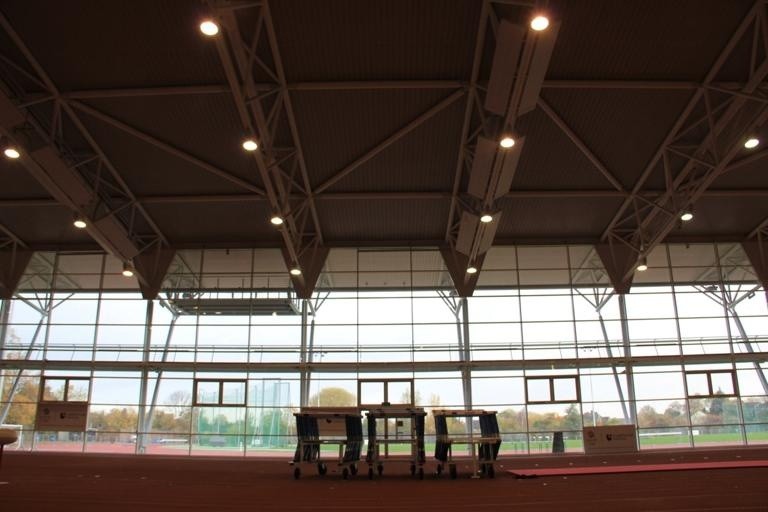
[292,411,504,481]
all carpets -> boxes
[505,459,768,477]
